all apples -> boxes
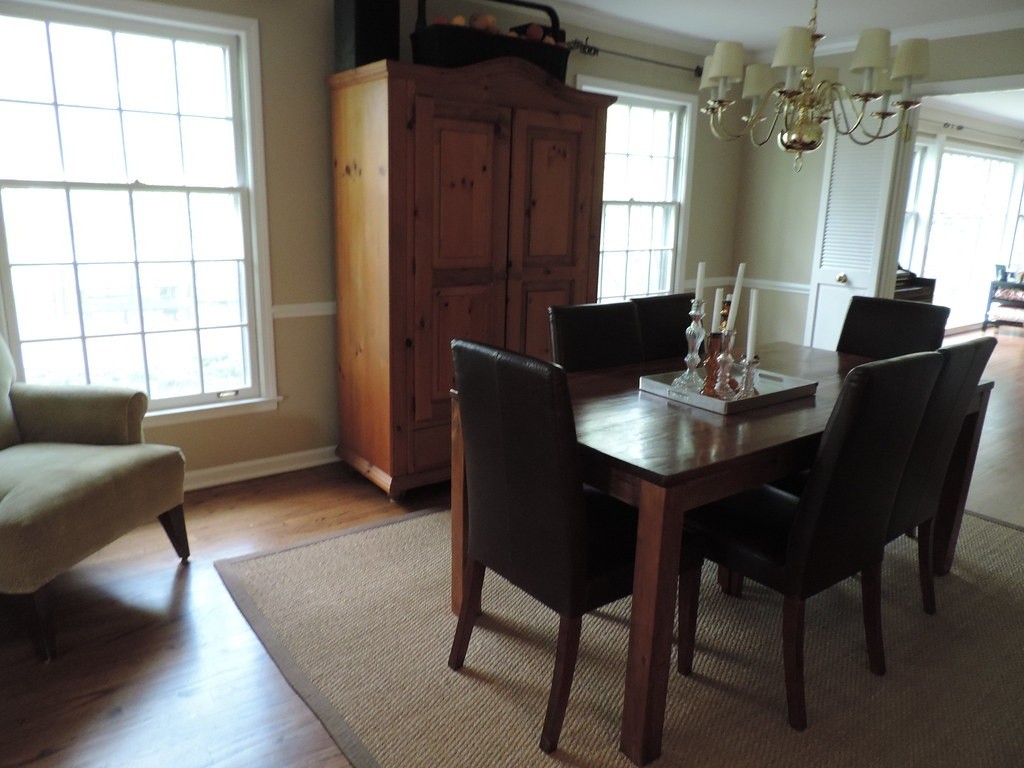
[431,13,555,46]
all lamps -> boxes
[699,0,931,173]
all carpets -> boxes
[212,503,1024,768]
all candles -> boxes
[728,294,732,299]
[711,287,725,332]
[725,262,746,328]
[746,287,760,360]
[696,262,707,299]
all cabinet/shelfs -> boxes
[329,58,618,509]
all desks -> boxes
[446,341,995,767]
[982,281,1024,331]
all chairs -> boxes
[836,295,950,359]
[633,290,695,363]
[678,351,944,730]
[0,333,191,660]
[447,338,720,753]
[546,300,636,373]
[764,337,999,616]
